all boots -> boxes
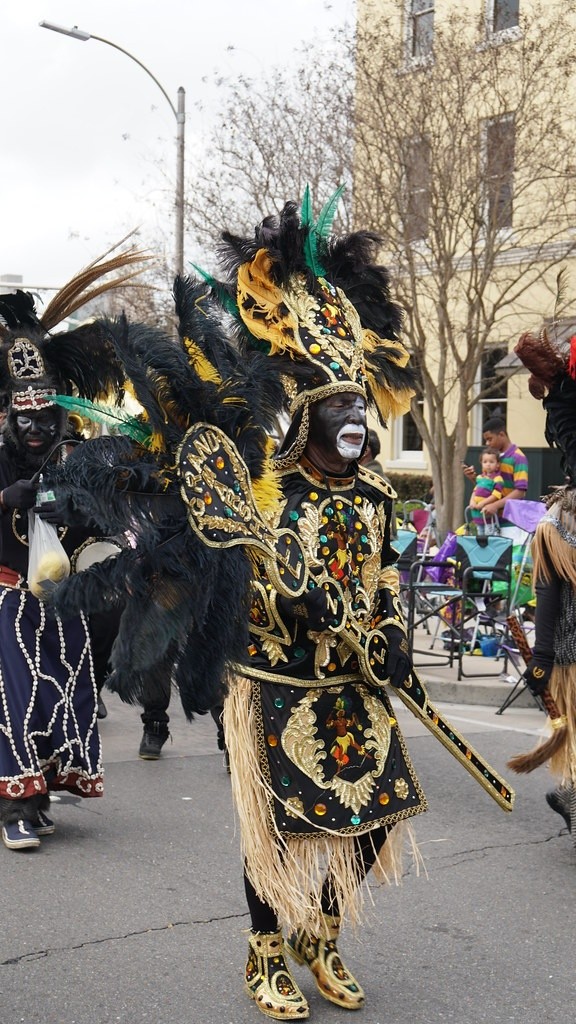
[243,923,309,1020]
[285,887,365,1010]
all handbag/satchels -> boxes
[27,495,70,601]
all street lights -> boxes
[39,17,187,277]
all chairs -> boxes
[386,500,516,681]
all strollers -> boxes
[401,498,438,613]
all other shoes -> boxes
[225,749,231,774]
[139,722,170,759]
[1,819,40,849]
[546,779,571,841]
[28,809,55,835]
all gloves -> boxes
[33,496,71,525]
[275,586,334,633]
[522,659,553,697]
[378,624,413,688]
[3,479,38,510]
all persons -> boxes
[0,394,231,848]
[215,379,429,1019]
[358,429,397,543]
[523,469,576,849]
[419,527,440,602]
[462,418,528,654]
[470,450,504,536]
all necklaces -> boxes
[316,464,358,581]
